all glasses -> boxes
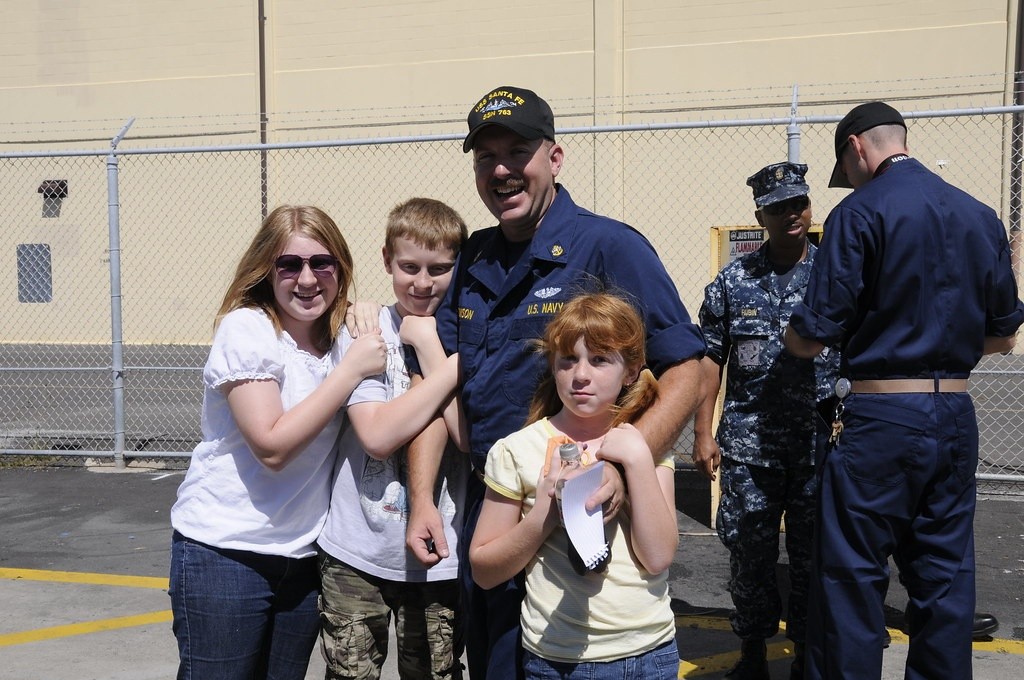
[276,253,336,278]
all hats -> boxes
[463,85,555,153]
[826,102,909,188]
[746,161,809,207]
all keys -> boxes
[829,402,845,446]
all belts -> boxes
[849,379,969,393]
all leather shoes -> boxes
[901,605,999,639]
[726,638,767,679]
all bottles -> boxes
[556,444,613,577]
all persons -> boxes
[783,100,1024,680]
[405,86,710,680]
[316,198,471,680]
[692,161,840,680]
[168,205,388,680]
[882,536,999,648]
[469,292,679,680]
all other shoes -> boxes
[883,628,891,646]
